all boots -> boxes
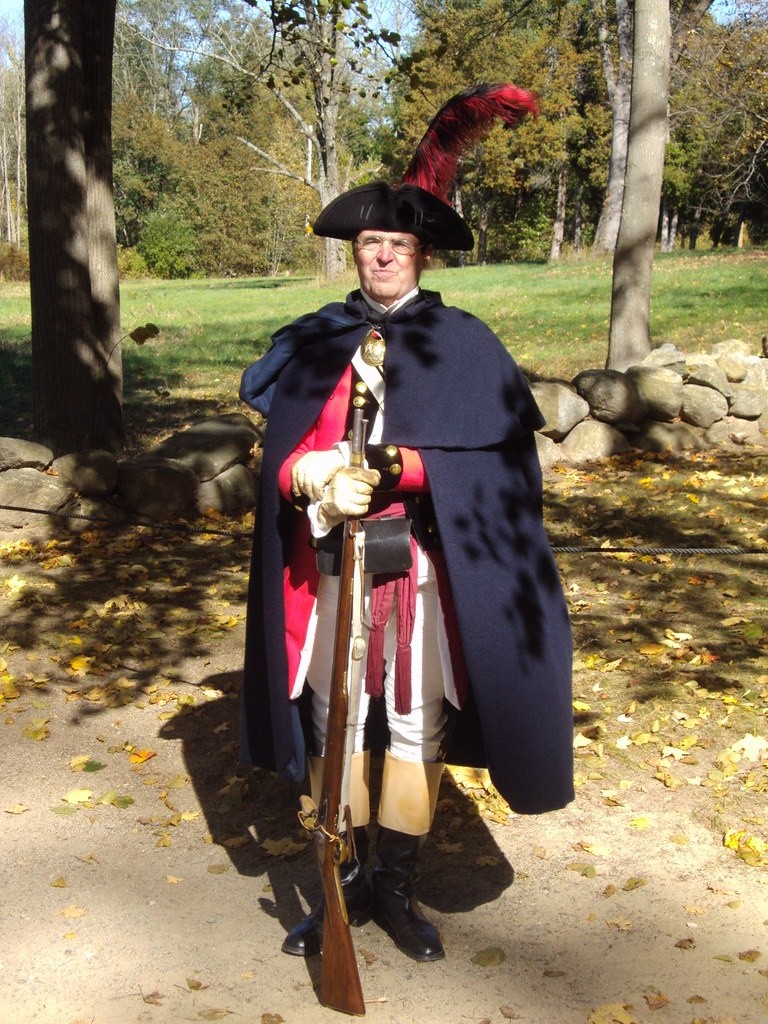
[284,748,373,952]
[368,746,447,959]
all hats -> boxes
[314,84,539,251]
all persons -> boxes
[238,183,574,961]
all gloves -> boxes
[306,467,381,539]
[291,440,354,501]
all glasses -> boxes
[354,235,422,256]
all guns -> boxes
[292,378,375,1018]
